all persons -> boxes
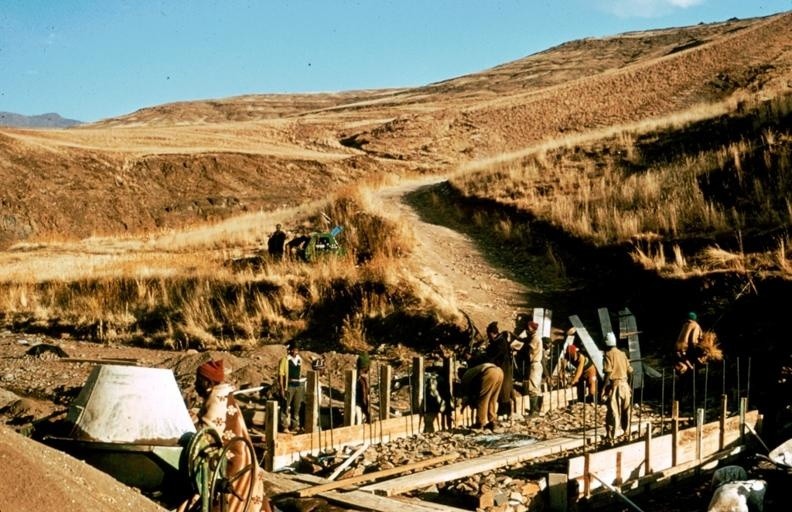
[674,311,705,369]
[193,359,272,512]
[354,350,370,426]
[267,223,286,261]
[304,359,326,425]
[457,313,599,435]
[278,338,304,433]
[600,332,634,448]
